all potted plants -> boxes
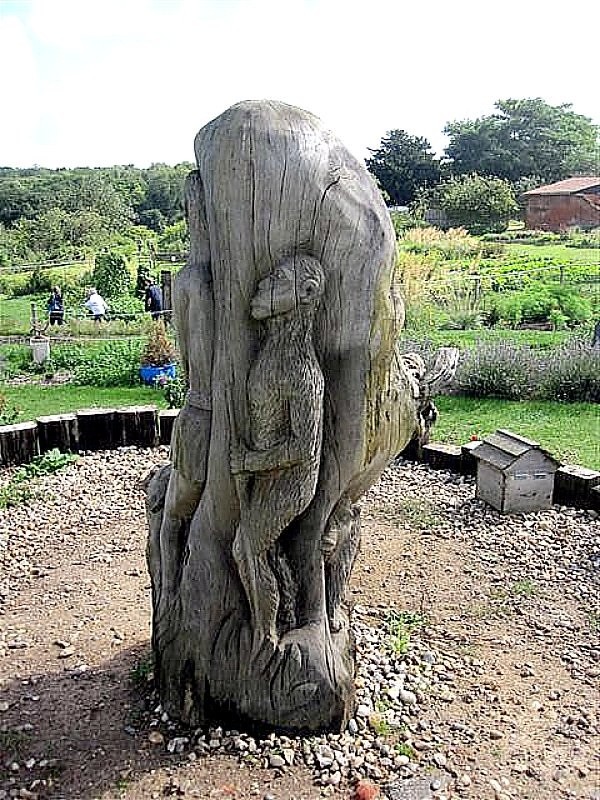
[139,336,176,384]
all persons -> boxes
[47,287,64,326]
[135,268,162,320]
[84,290,109,321]
